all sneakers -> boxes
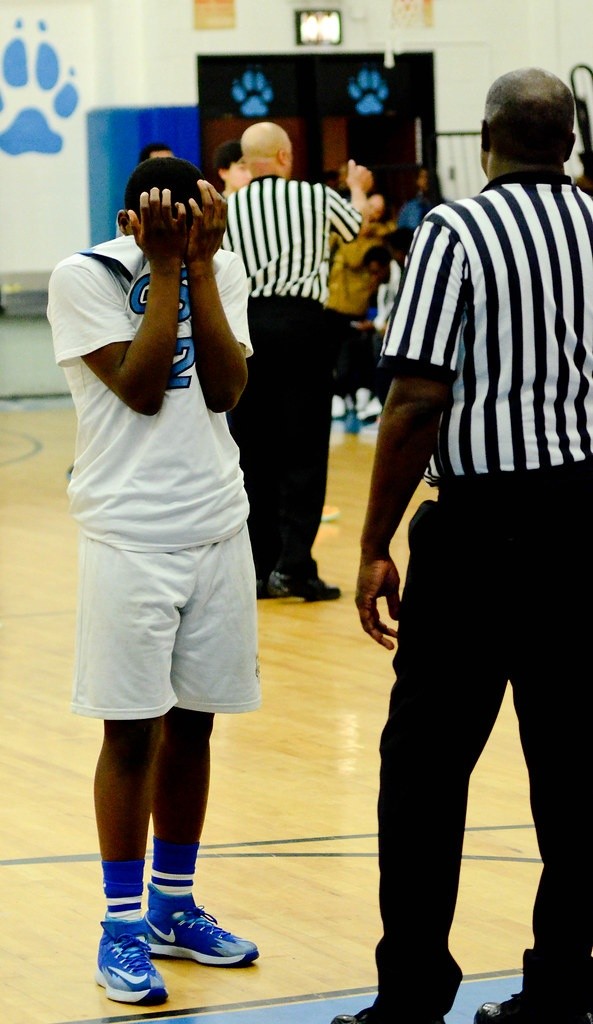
[143,883,259,968]
[95,920,169,1006]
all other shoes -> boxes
[320,506,341,521]
[256,560,340,602]
[331,1007,371,1024]
[473,1002,501,1024]
[332,386,382,421]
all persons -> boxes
[45,156,260,1003]
[332,66,593,1024]
[136,121,441,603]
